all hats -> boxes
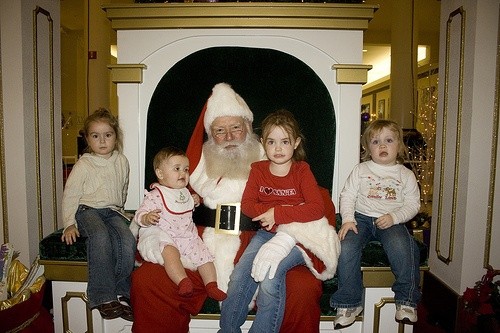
[186,83,254,190]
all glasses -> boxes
[210,117,245,140]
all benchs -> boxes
[40,221,428,332]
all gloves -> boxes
[138,225,178,266]
[251,231,295,282]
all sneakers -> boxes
[334,305,365,329]
[395,303,417,325]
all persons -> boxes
[217,110,324,333]
[330,119,420,330]
[62,107,136,322]
[134,149,227,302]
[130,83,342,333]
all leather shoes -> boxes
[117,296,135,321]
[98,301,124,319]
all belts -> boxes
[192,201,263,235]
